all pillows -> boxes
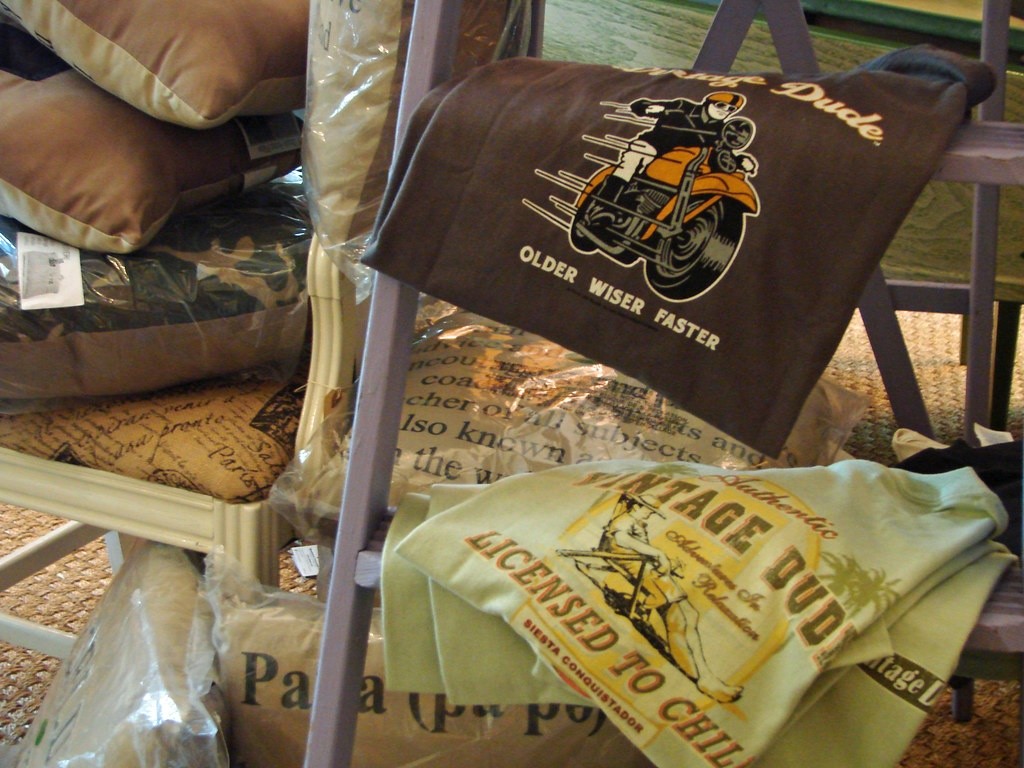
[0,0,313,401]
[296,307,865,533]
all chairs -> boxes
[0,0,543,661]
